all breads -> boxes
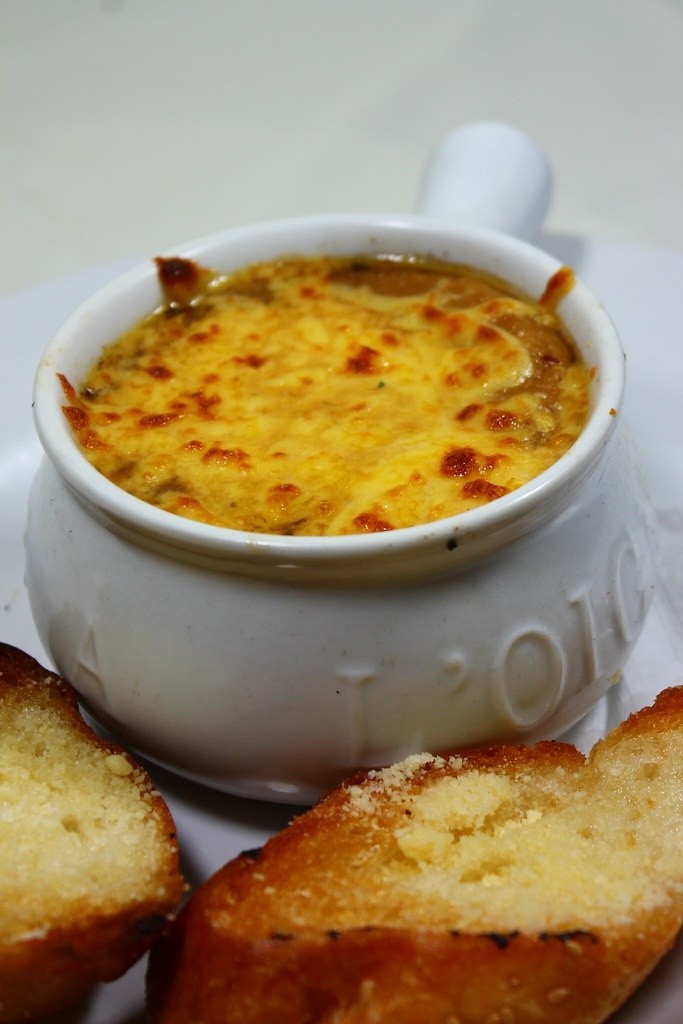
[144,688,683,1024]
[0,642,182,1024]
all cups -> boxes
[24,121,663,808]
